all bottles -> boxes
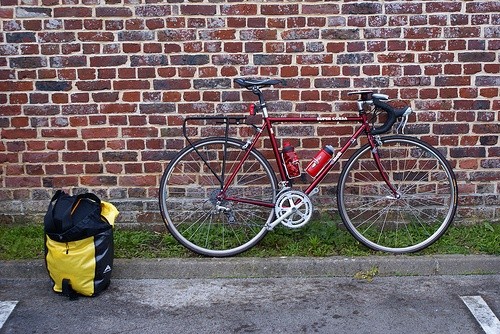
[304,144,337,177]
[281,140,301,178]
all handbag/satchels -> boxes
[44,190,120,301]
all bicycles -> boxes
[158,77,460,257]
